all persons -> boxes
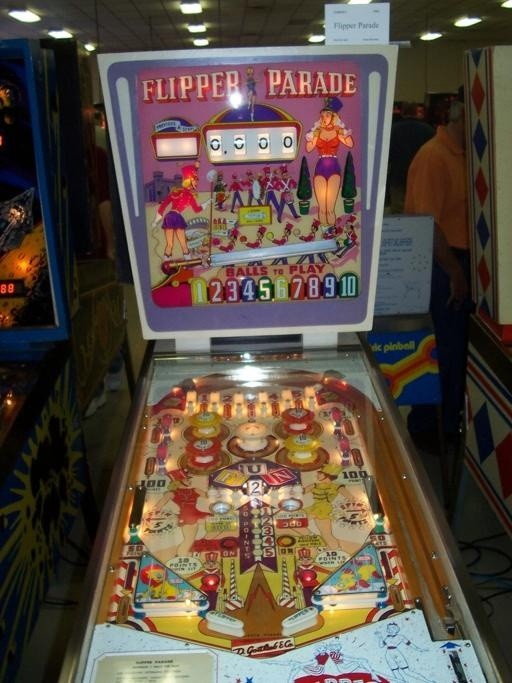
[186,562,226,610]
[384,85,469,528]
[262,622,436,683]
[81,103,123,419]
[303,470,355,551]
[155,479,214,558]
[293,558,332,607]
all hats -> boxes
[320,96,343,114]
[181,165,197,180]
